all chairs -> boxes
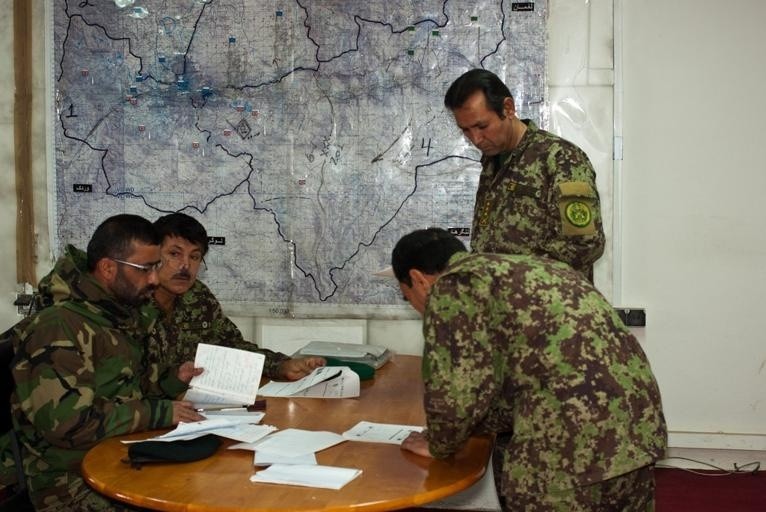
[1,336,36,511]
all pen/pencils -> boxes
[193,408,204,412]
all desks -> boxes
[81,355,489,512]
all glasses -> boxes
[107,255,164,274]
[161,253,208,277]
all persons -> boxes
[8,211,205,510]
[140,213,329,512]
[389,226,666,511]
[445,70,604,287]
